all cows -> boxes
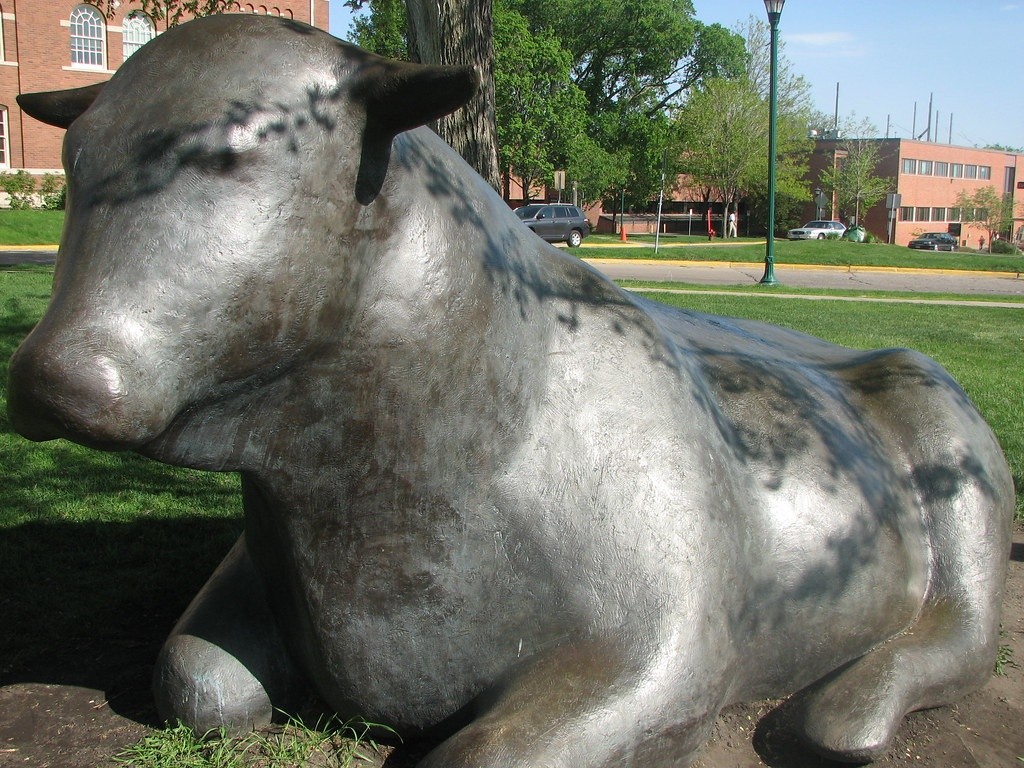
[15,14,1015,767]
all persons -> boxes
[727,211,738,238]
[975,230,1001,250]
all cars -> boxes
[787,219,846,242]
[908,232,957,251]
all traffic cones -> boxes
[621,224,628,241]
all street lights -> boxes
[814,186,821,221]
[750,1,787,284]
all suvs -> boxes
[513,203,592,248]
